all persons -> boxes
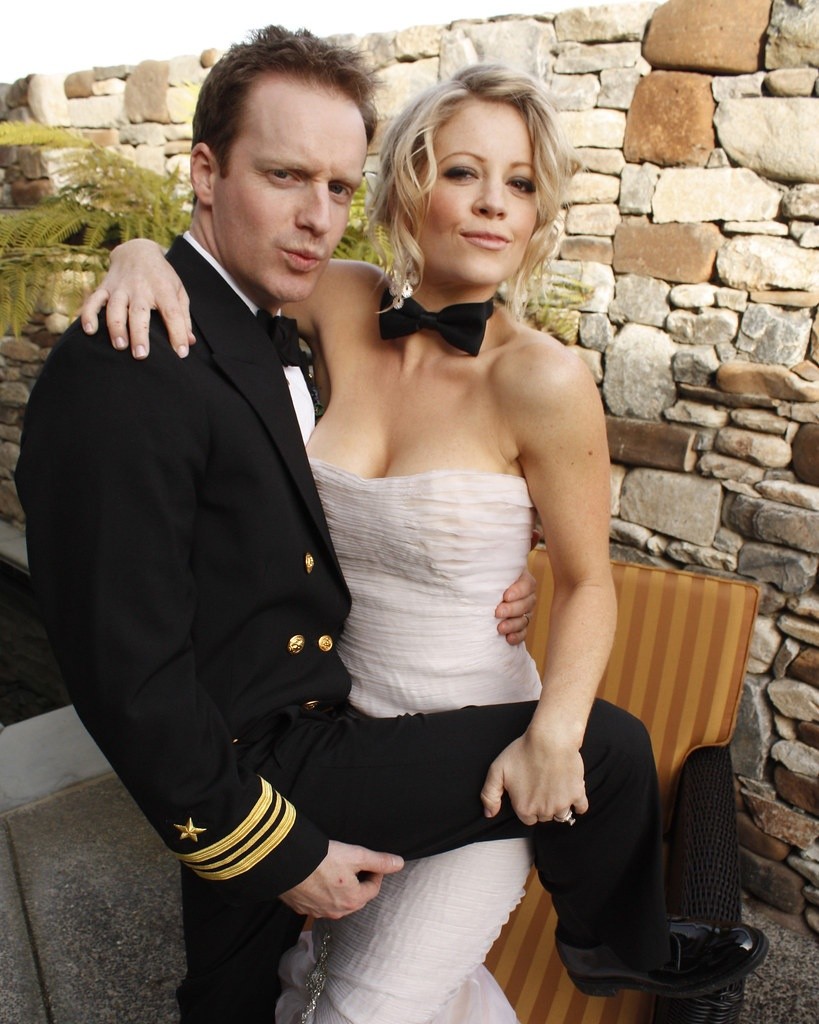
[76,63,621,1024]
[14,18,769,1023]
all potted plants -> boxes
[0,118,192,340]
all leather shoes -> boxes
[556,915,769,997]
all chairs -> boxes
[478,549,759,1023]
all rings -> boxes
[553,809,577,826]
[523,614,529,627]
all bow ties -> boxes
[379,287,505,357]
[256,308,301,367]
